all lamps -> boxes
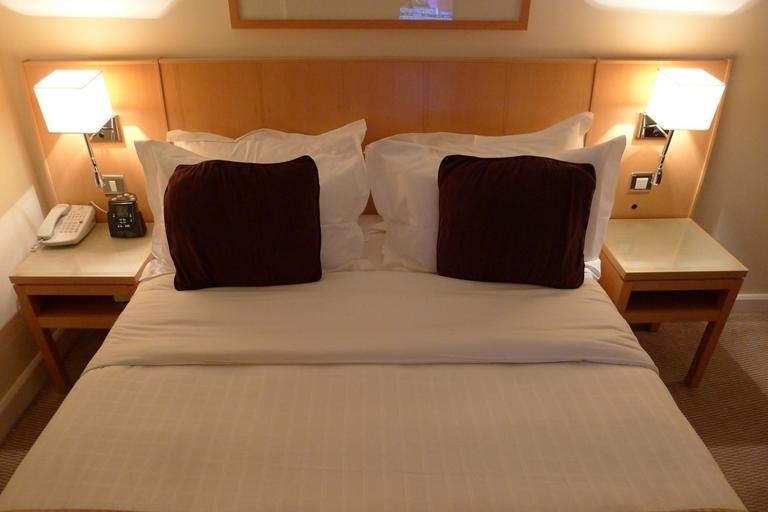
[636,66,726,185]
[34,69,123,189]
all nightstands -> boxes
[8,222,154,395]
[597,217,749,390]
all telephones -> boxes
[29,203,97,251]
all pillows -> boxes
[369,136,626,289]
[134,139,364,281]
[365,111,594,233]
[166,118,370,220]
[164,156,322,291]
[436,155,596,289]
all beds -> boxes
[0,56,747,512]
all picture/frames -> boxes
[227,0,530,30]
